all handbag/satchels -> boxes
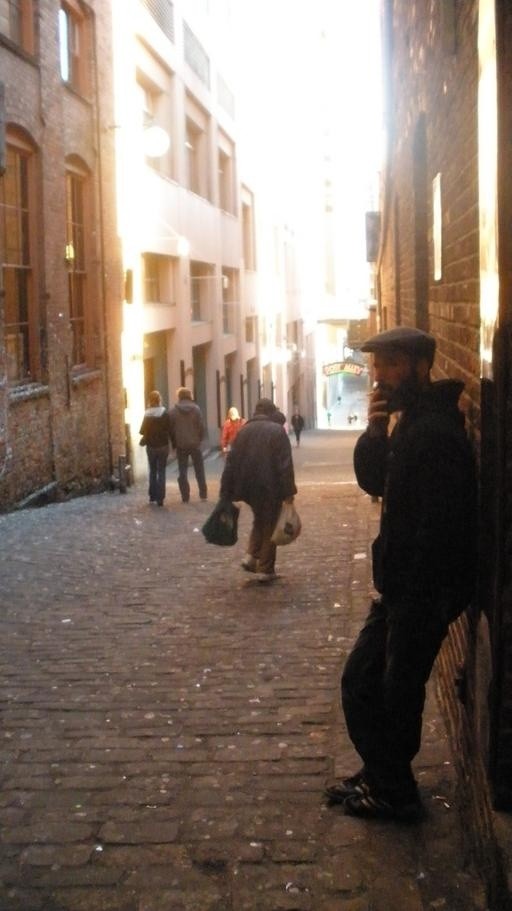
[202,498,239,545]
[270,498,302,545]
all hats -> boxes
[255,398,276,413]
[361,325,436,358]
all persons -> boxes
[291,409,304,448]
[139,391,177,508]
[219,399,298,584]
[322,328,480,819]
[167,387,208,503]
[221,407,247,462]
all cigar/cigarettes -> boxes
[365,393,374,397]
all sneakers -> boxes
[258,571,280,582]
[321,773,373,800]
[241,553,257,573]
[342,791,423,820]
